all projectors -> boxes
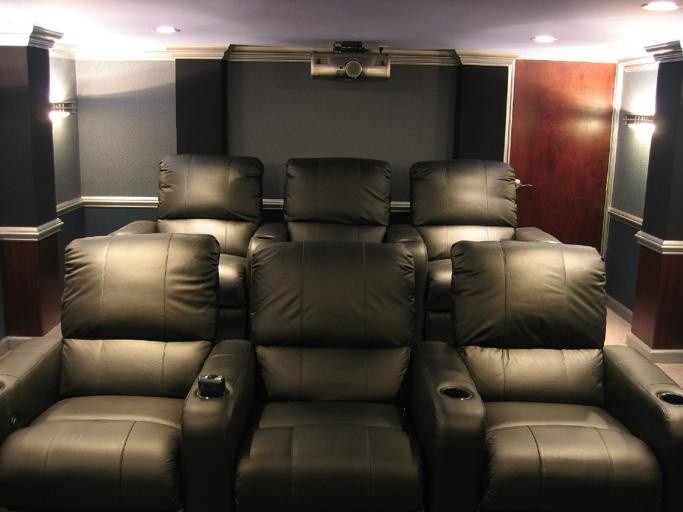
[310,53,391,79]
